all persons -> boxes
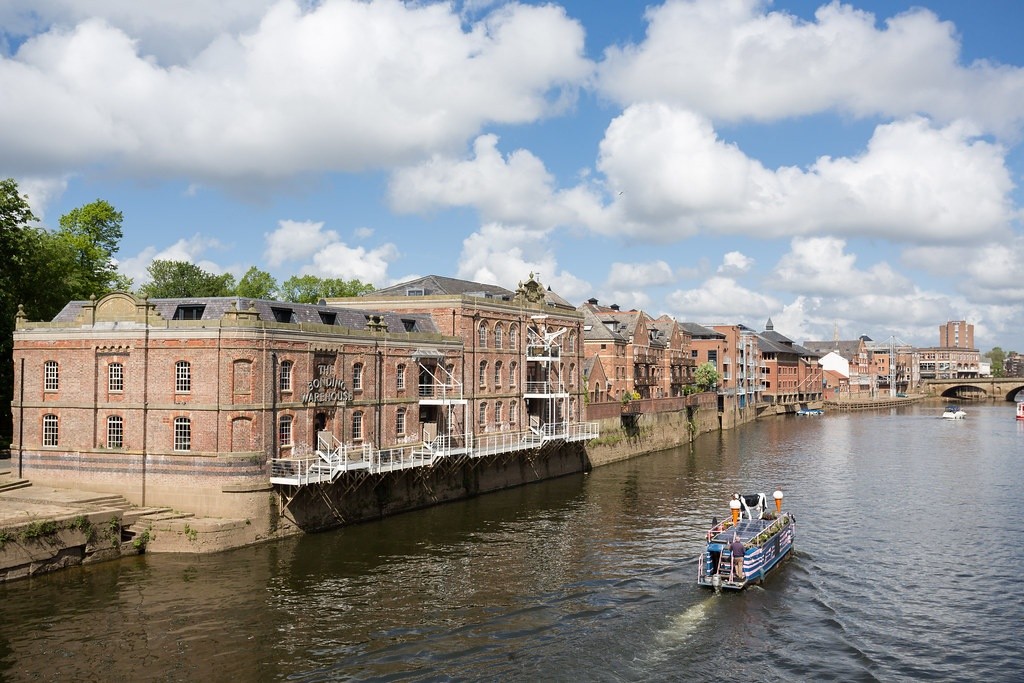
[729,493,742,518]
[728,536,746,579]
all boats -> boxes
[1016,400,1024,419]
[943,405,967,419]
[794,408,824,417]
[696,490,796,594]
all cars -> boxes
[896,393,908,398]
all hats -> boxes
[734,537,740,541]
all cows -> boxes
[732,492,768,521]
